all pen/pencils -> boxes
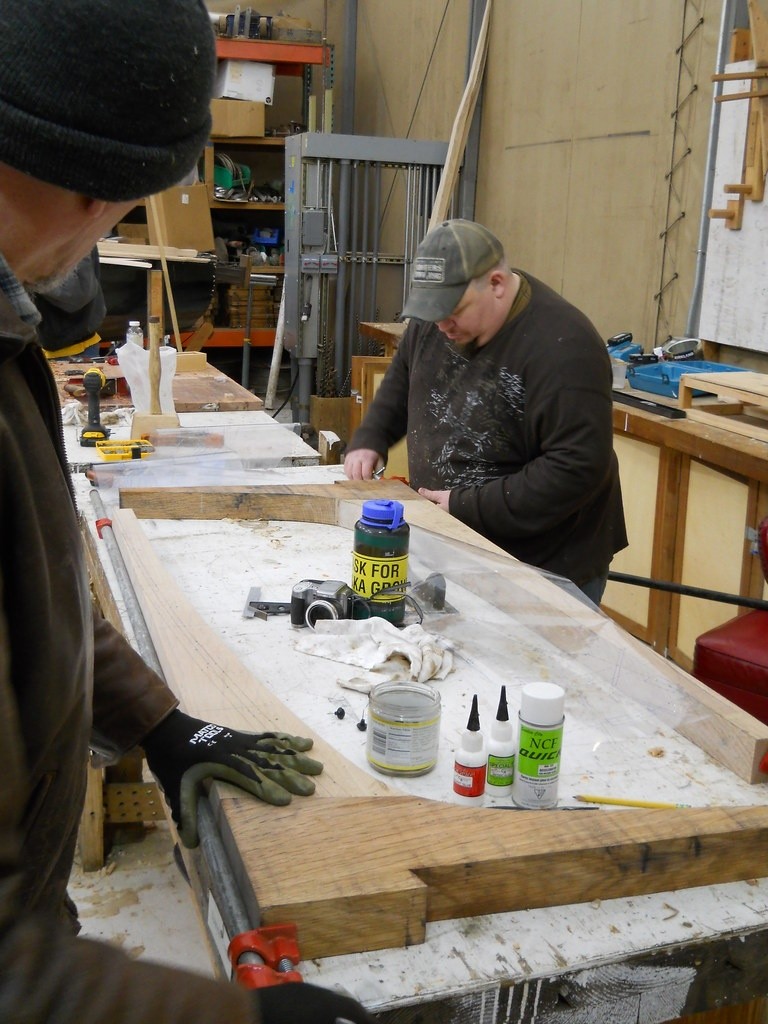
[573,795,678,808]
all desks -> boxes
[49,350,270,415]
[64,407,322,470]
[350,319,768,672]
[68,459,768,1024]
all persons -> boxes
[342,218,628,611]
[0,0,370,1024]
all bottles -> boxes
[513,683,566,809]
[454,694,487,797]
[126,321,143,351]
[352,498,410,626]
[485,685,516,797]
[364,682,441,777]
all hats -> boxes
[400,219,507,319]
[0,1,217,202]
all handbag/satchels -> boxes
[115,338,175,416]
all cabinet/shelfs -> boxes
[96,36,335,349]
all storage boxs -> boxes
[116,223,149,245]
[206,98,268,140]
[215,58,275,105]
[177,351,210,371]
[149,183,217,254]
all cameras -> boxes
[291,579,355,631]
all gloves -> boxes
[257,980,379,1024]
[142,710,323,849]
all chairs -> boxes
[691,513,768,719]
